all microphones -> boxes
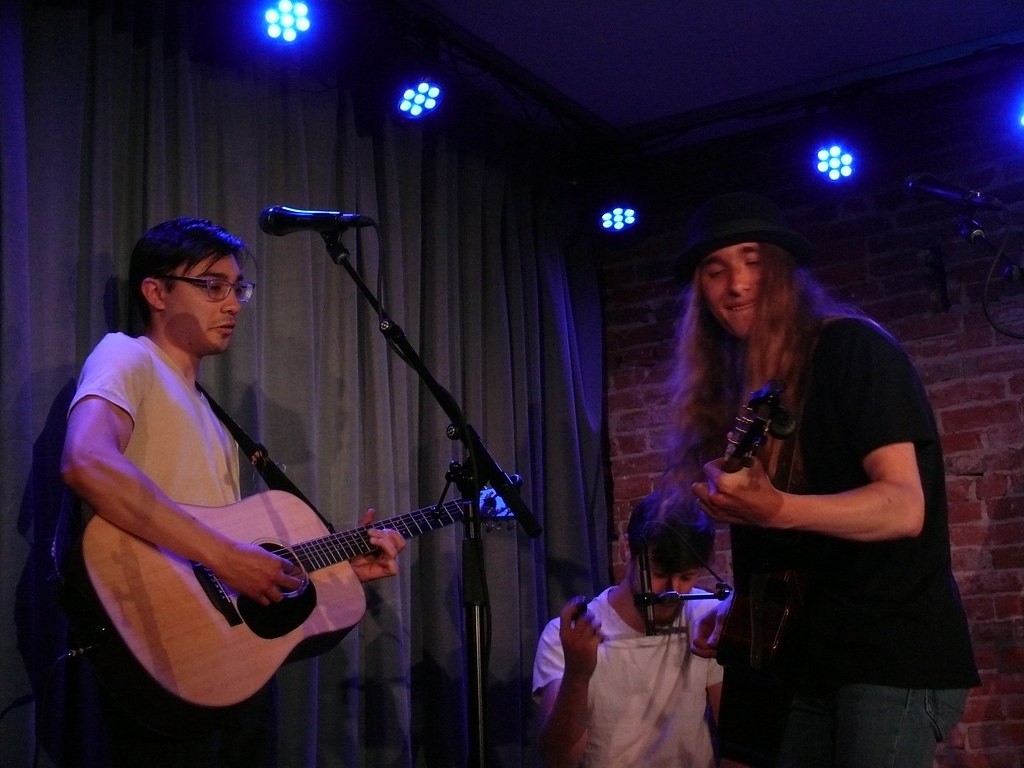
[258,205,372,238]
[640,541,656,636]
[904,170,1006,215]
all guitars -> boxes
[74,481,520,727]
[711,370,807,766]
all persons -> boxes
[663,188,984,768]
[531,486,723,768]
[61,218,408,768]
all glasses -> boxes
[155,275,255,303]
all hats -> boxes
[671,193,810,264]
